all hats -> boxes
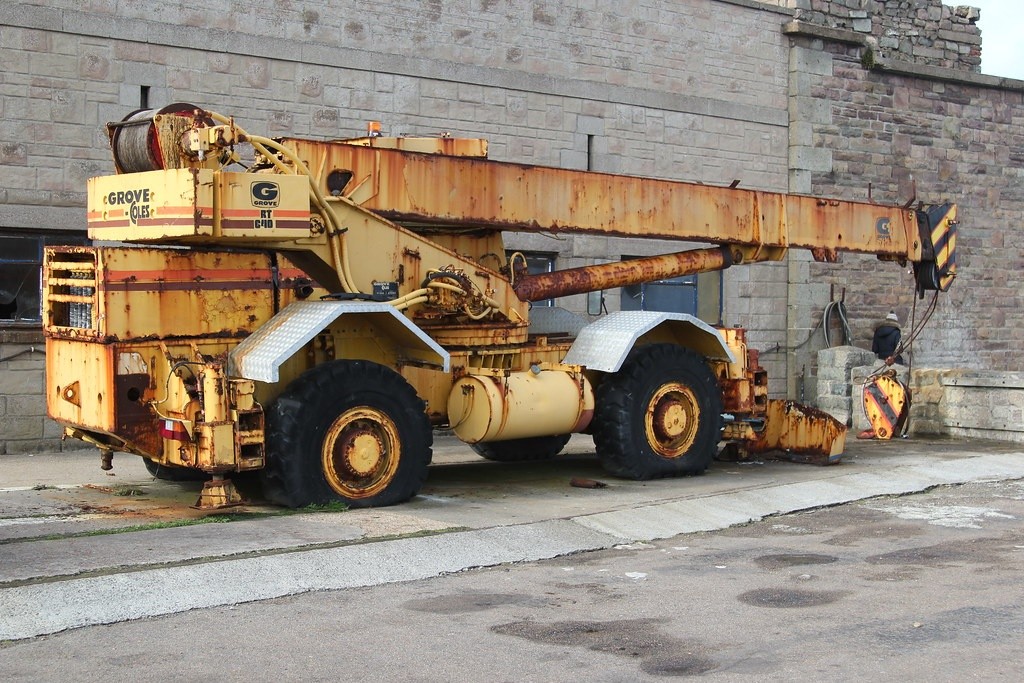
[886,311,898,322]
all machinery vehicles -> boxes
[39,81,961,512]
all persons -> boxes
[872,311,904,365]
